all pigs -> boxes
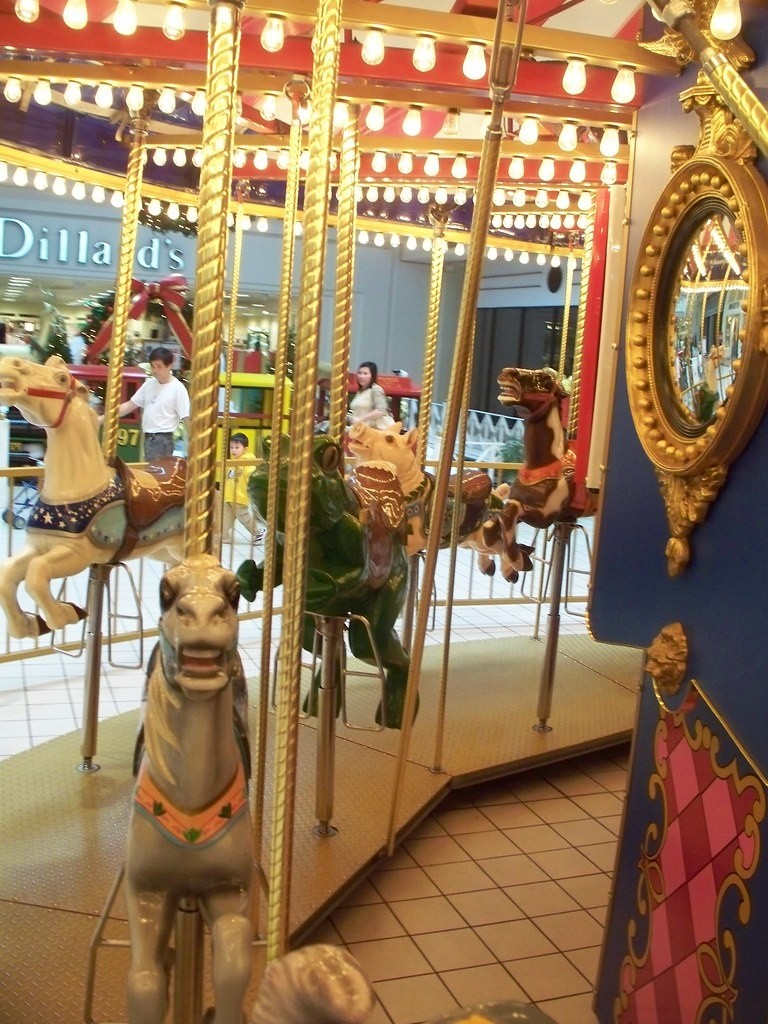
[345,421,521,584]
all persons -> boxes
[344,361,387,433]
[213,432,266,546]
[96,347,191,462]
[254,342,262,359]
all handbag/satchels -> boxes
[375,415,395,429]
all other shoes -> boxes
[222,538,231,544]
[254,529,266,546]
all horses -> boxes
[121,553,257,1023]
[1,353,263,641]
[691,344,727,421]
[476,365,600,572]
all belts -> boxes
[143,432,174,437]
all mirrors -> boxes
[623,60,768,578]
